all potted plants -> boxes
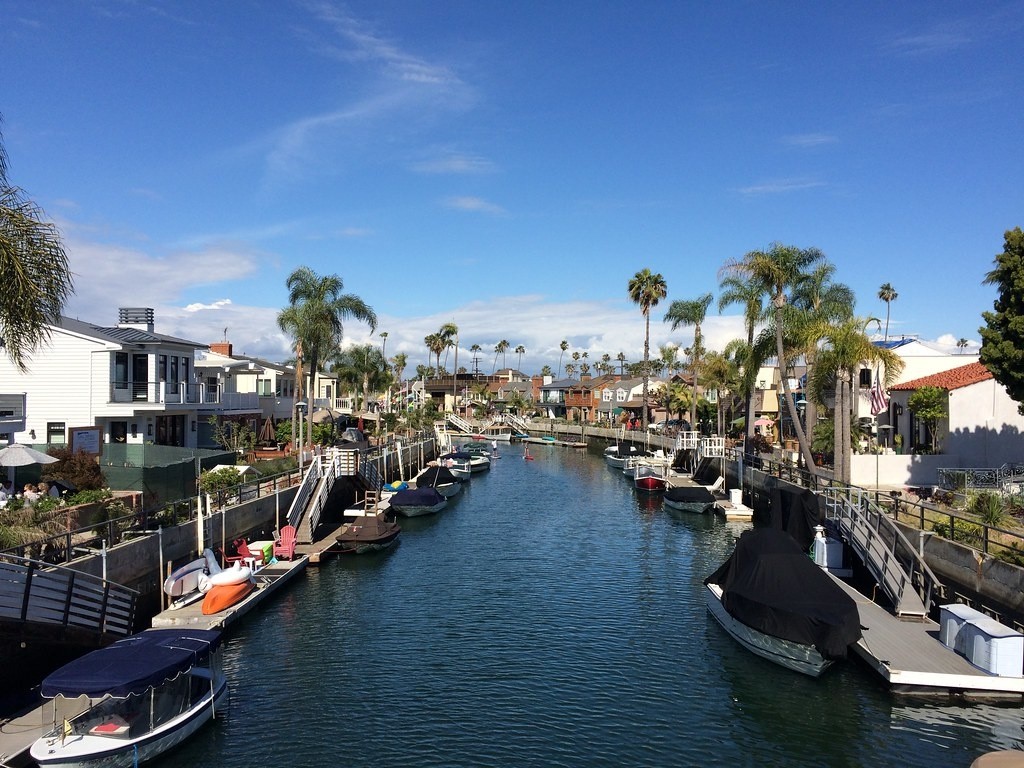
[784,434,799,452]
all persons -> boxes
[22,482,49,500]
[627,410,634,419]
[635,419,640,430]
[626,420,632,431]
[0,480,15,501]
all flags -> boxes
[461,386,469,394]
[869,372,888,415]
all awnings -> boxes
[618,401,643,407]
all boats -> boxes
[603,425,717,515]
[334,432,493,556]
[27,620,232,768]
[702,525,862,679]
[164,547,259,615]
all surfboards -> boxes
[490,456,502,459]
[520,455,533,460]
[164,557,207,610]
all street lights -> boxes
[796,399,809,469]
[295,401,309,481]
[878,424,895,455]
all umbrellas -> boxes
[303,408,347,424]
[355,411,384,421]
[258,417,275,447]
[730,415,760,424]
[0,442,60,467]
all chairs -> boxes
[272,525,297,562]
[233,537,265,569]
[219,547,246,570]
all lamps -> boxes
[29,430,35,436]
[148,424,153,436]
[198,372,203,377]
[227,374,232,378]
[193,420,197,432]
[897,402,903,416]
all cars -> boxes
[662,418,691,440]
[648,420,666,435]
